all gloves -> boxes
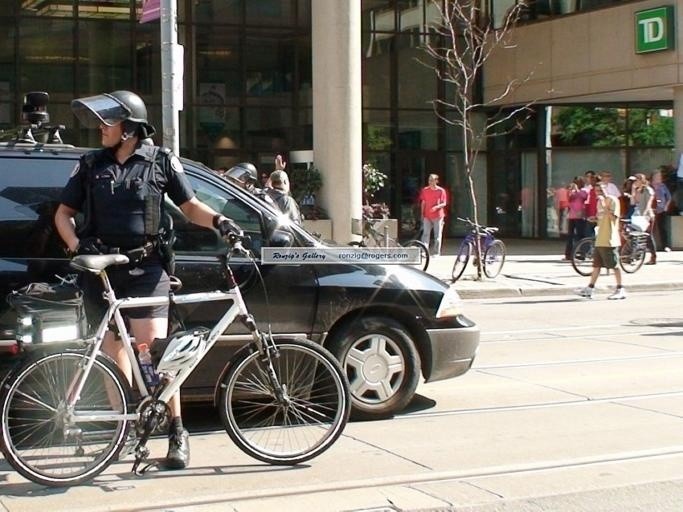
[73,239,107,254]
[218,217,245,242]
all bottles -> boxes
[135,342,158,388]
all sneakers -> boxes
[166,427,188,469]
[574,287,593,297]
[608,288,625,299]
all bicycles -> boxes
[453,216,507,282]
[348,215,430,275]
[0,218,351,489]
[573,220,651,277]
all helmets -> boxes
[151,324,211,379]
[223,163,257,183]
[71,91,149,129]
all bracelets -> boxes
[211,214,225,229]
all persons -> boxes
[575,182,627,300]
[555,183,568,232]
[417,173,446,255]
[561,163,672,267]
[213,154,303,225]
[52,90,242,469]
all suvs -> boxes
[0,90,479,442]
